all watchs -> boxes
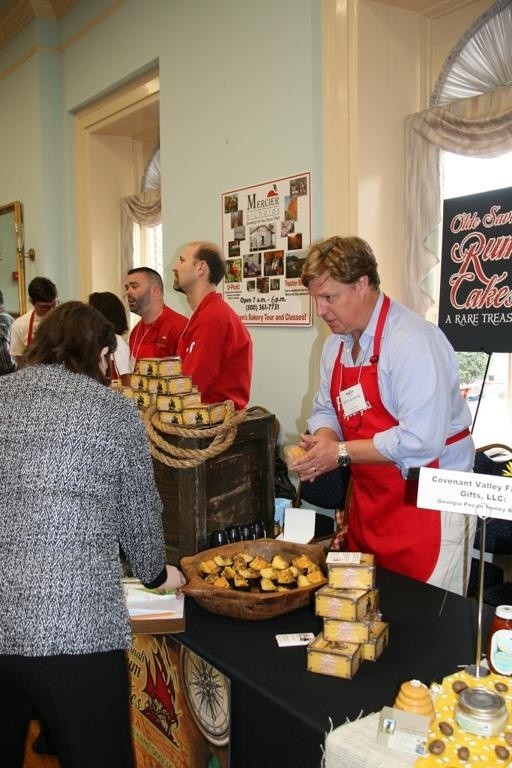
[336,439,351,466]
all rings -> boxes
[310,465,318,472]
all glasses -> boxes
[36,299,60,311]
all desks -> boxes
[162,546,512,767]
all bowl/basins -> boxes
[179,538,330,618]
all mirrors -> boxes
[0,199,27,320]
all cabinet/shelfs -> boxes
[152,409,276,563]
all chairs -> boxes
[286,462,350,550]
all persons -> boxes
[9,276,64,371]
[87,290,136,388]
[125,267,191,376]
[282,232,480,597]
[172,241,254,409]
[0,299,189,767]
[0,291,19,377]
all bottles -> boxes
[211,523,266,547]
[486,603,512,677]
